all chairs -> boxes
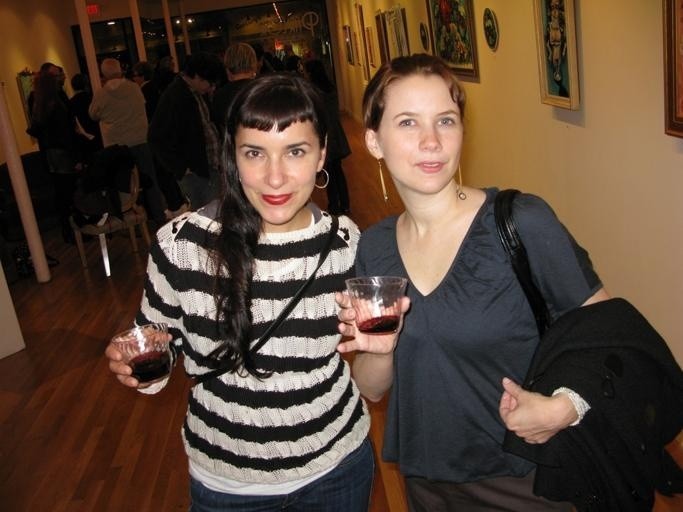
[72,164,153,277]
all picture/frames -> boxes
[426,0,480,84]
[662,0,683,137]
[16,71,40,144]
[342,4,411,85]
[532,0,582,110]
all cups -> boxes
[111,322,172,385]
[345,275,409,337]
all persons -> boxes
[26,41,350,218]
[332,54,609,511]
[106,75,376,512]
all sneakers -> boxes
[164,204,186,219]
